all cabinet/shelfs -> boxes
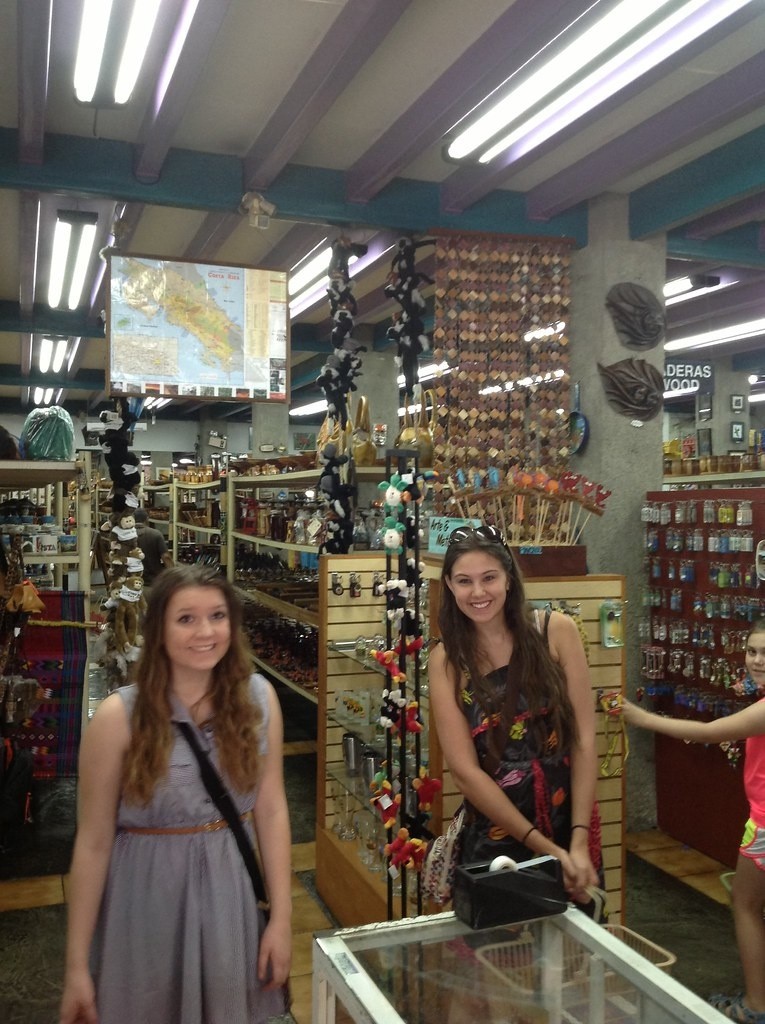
[1,451,628,929]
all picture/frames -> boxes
[730,394,746,411]
[730,420,745,441]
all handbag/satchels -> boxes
[282,980,295,1013]
[420,803,475,908]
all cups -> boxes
[0,514,78,556]
[334,784,427,918]
[363,750,384,791]
[341,734,361,773]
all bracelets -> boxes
[521,827,538,846]
[569,822,593,832]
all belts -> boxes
[120,812,253,835]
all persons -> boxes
[427,526,607,1024]
[94,509,175,588]
[621,619,765,1024]
[60,565,296,1024]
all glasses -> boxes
[447,525,504,544]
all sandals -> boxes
[708,992,765,1024]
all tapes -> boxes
[489,855,518,872]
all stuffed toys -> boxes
[318,237,366,553]
[101,395,144,686]
[369,235,443,875]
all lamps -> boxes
[26,1,765,422]
